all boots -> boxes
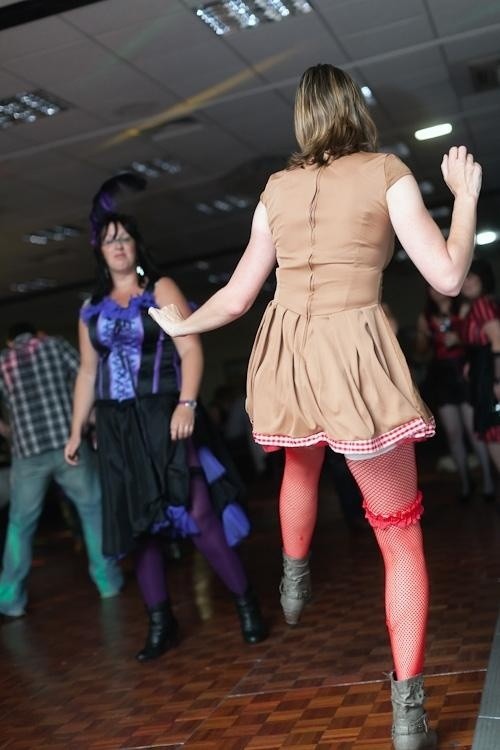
[279,550,312,624]
[135,600,178,663]
[389,671,438,750]
[231,584,268,645]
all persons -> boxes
[416,263,500,497]
[1,320,130,620]
[142,62,482,750]
[62,212,264,662]
